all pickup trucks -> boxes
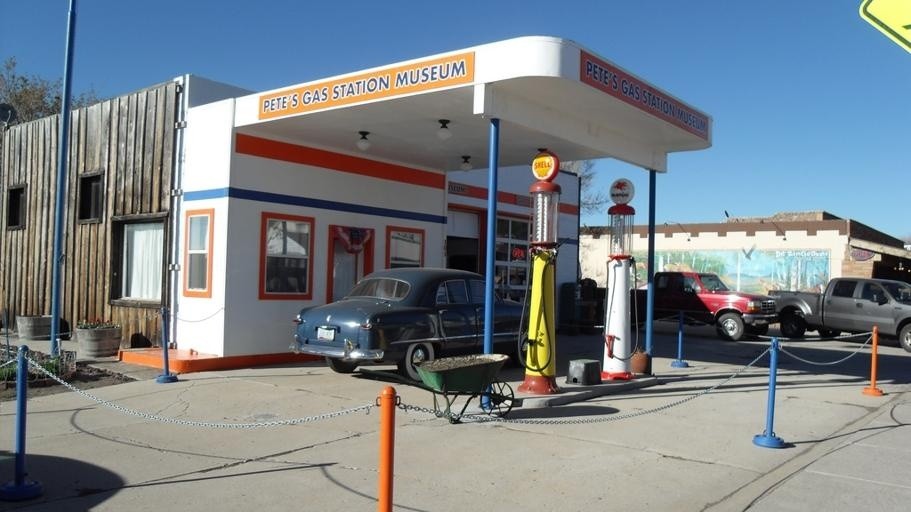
[769,277,911,352]
[596,271,779,341]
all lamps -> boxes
[355,129,371,151]
[437,117,473,171]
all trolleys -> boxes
[352,352,515,424]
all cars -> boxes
[290,267,530,374]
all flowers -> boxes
[76,318,121,330]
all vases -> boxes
[77,328,123,358]
[15,314,52,341]
